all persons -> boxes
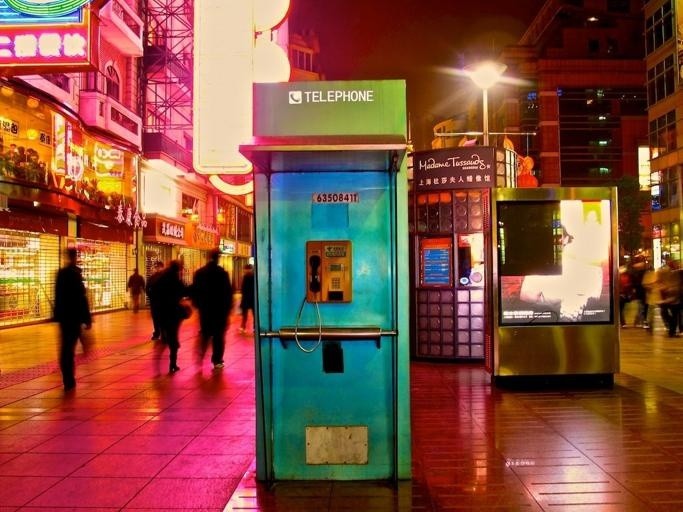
[146,261,166,344]
[126,268,145,312]
[619,255,683,335]
[50,244,93,392]
[192,247,234,369]
[149,258,192,372]
[239,264,255,334]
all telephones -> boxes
[306,240,352,303]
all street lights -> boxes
[465,62,508,147]
